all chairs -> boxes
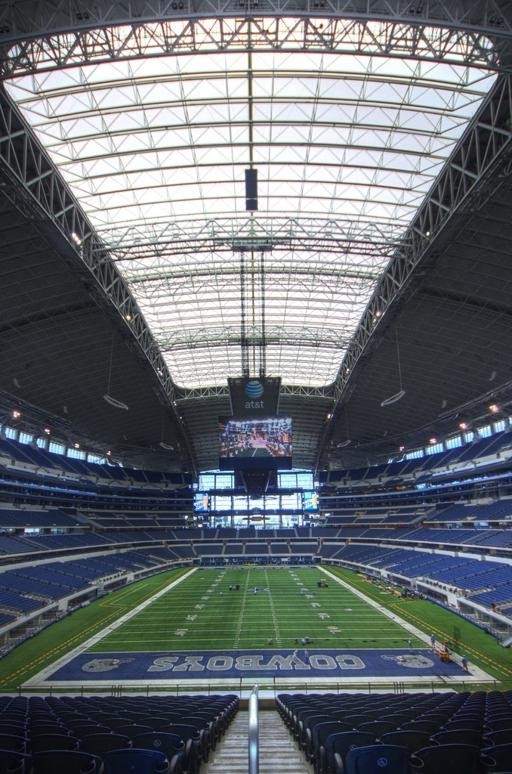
[0,695,237,774]
[277,692,512,772]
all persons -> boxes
[408,638,413,652]
[431,634,436,648]
[462,655,468,672]
